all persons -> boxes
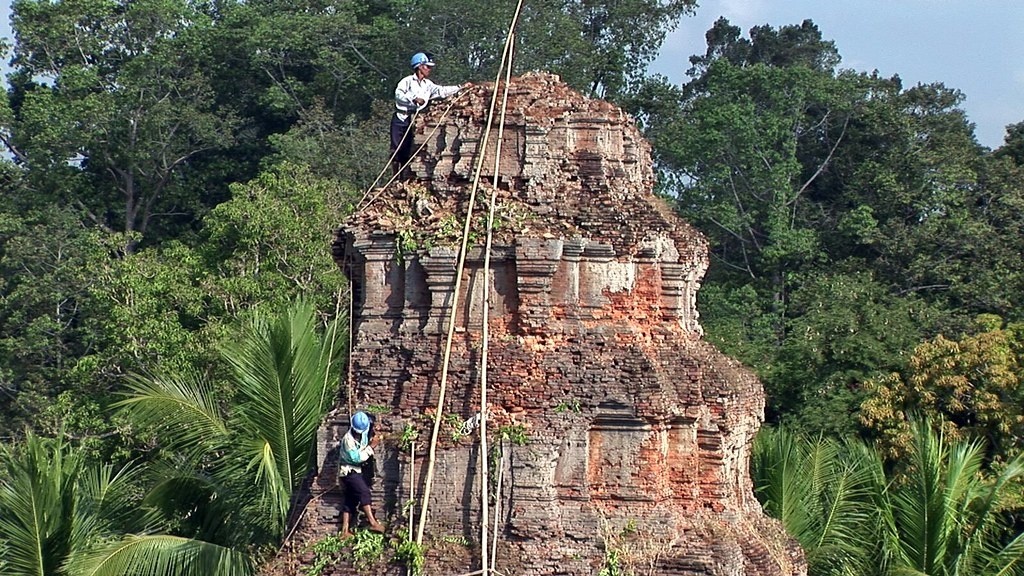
[336,412,386,539]
[389,52,472,180]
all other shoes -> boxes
[369,525,385,534]
[339,533,353,540]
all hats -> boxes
[411,52,436,69]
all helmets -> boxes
[351,412,370,433]
